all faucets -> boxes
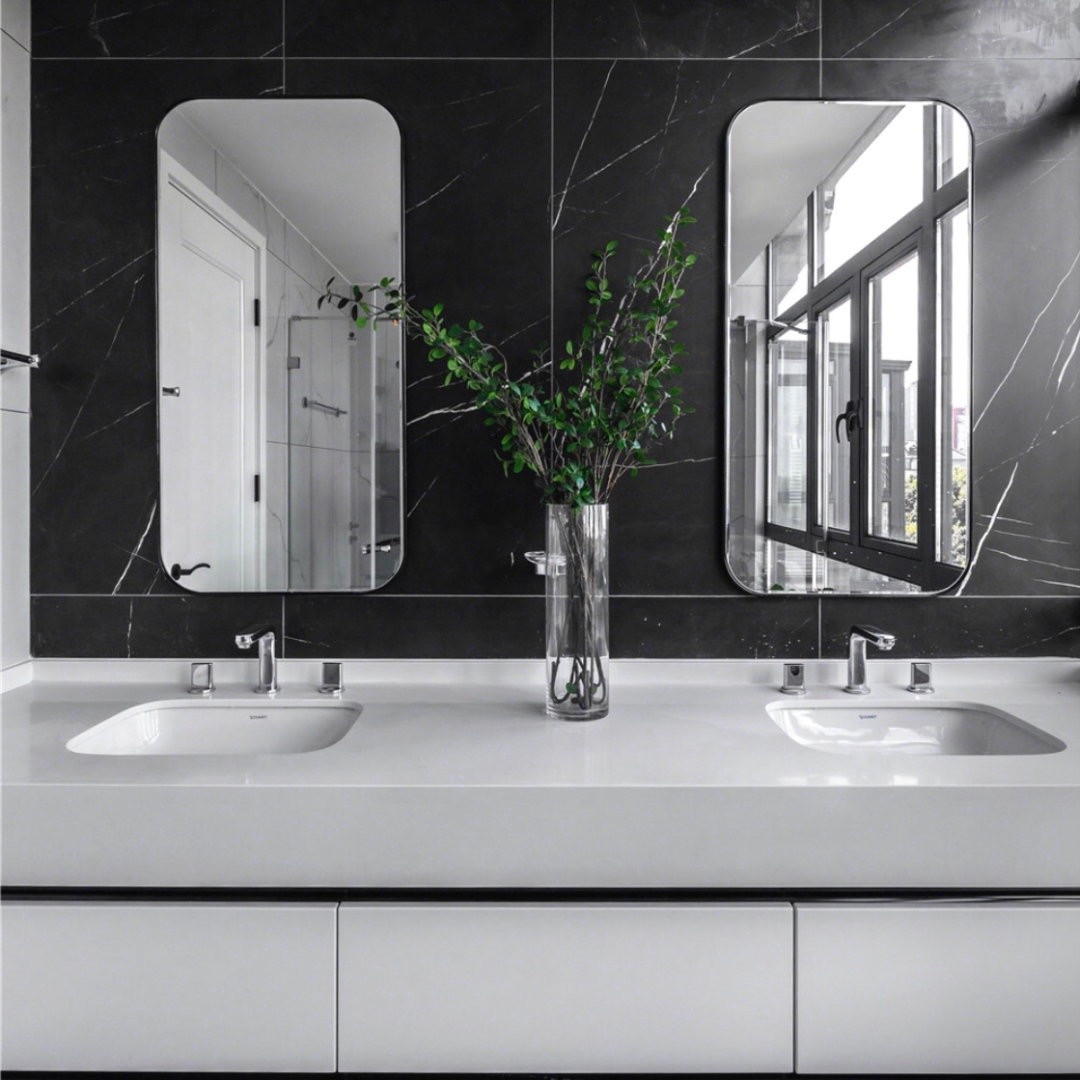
[841,625,897,695]
[234,622,281,694]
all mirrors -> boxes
[717,96,981,596]
[151,98,406,597]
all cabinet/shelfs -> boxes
[0,881,1080,1080]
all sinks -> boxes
[61,692,366,761]
[763,699,1070,760]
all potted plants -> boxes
[314,158,718,726]
